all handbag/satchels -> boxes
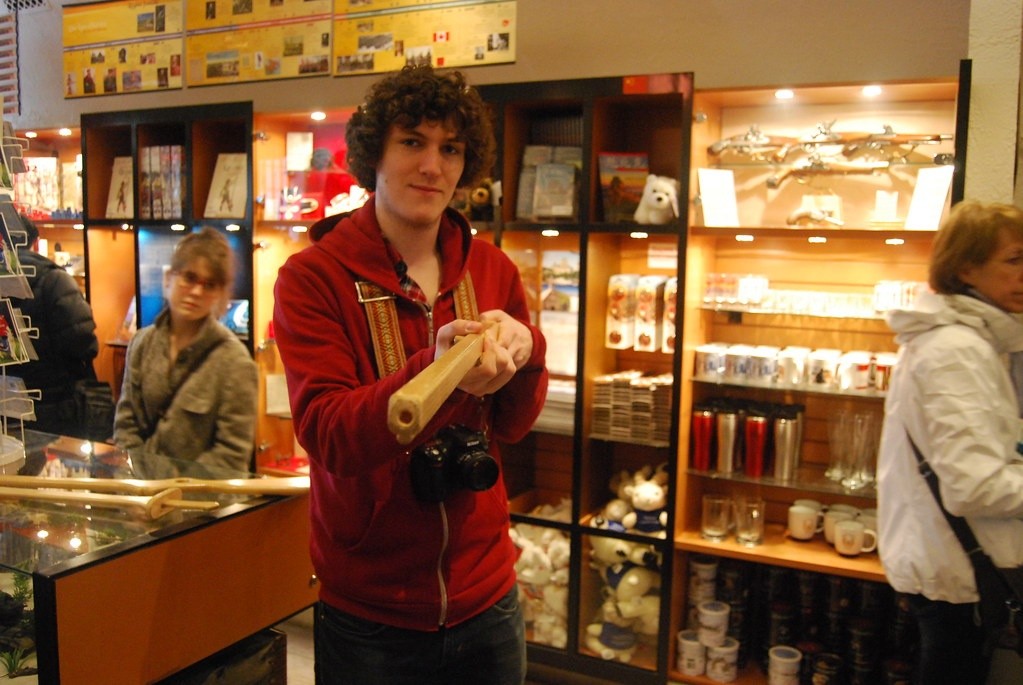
[970,554,1022,652]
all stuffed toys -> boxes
[468,178,493,222]
[584,535,662,661]
[633,174,680,225]
[589,465,667,530]
[510,522,570,648]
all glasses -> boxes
[175,269,222,297]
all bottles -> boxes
[676,559,927,684]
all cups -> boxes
[694,337,898,392]
[786,407,882,554]
[701,489,766,542]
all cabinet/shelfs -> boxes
[17,58,975,685]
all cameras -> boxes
[412,424,499,496]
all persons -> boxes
[395,42,403,57]
[607,176,625,223]
[158,68,167,87]
[219,180,233,210]
[119,48,126,63]
[299,57,328,74]
[104,69,116,92]
[0,210,99,436]
[116,180,126,214]
[112,226,257,480]
[84,68,95,94]
[272,60,548,685]
[171,56,180,76]
[878,206,1023,685]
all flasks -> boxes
[692,393,806,482]
